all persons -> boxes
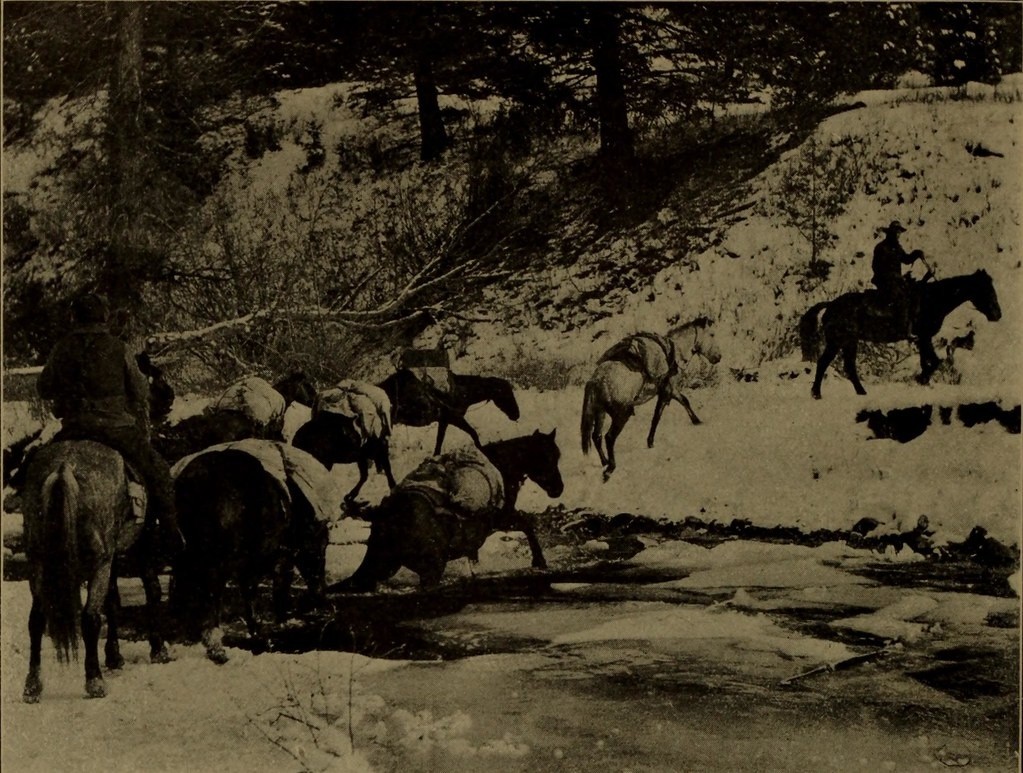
[35,294,186,549]
[870,220,924,342]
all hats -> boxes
[71,294,106,324]
[881,221,907,233]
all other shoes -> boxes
[160,525,186,551]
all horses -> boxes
[799,269,1002,401]
[18,350,562,703]
[582,317,722,483]
